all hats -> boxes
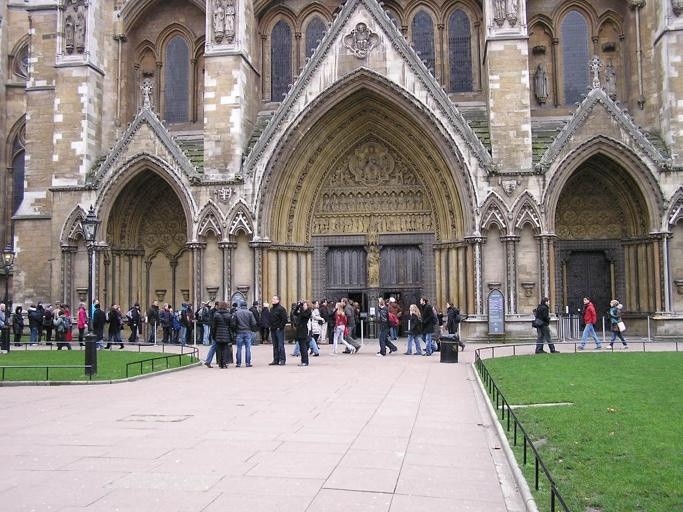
[93,304,101,308]
[232,301,269,307]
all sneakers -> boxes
[535,343,632,354]
[0,337,189,353]
[203,337,468,371]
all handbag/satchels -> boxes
[344,326,349,336]
[610,321,627,334]
[126,310,133,319]
[532,319,545,329]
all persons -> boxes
[377,297,402,356]
[576,296,602,350]
[148,300,159,343]
[125,304,145,342]
[92,299,125,349]
[290,297,361,365]
[404,297,465,356]
[0,297,89,350]
[159,296,288,369]
[604,299,629,349]
[534,297,560,354]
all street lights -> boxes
[75,204,103,378]
[0,243,18,356]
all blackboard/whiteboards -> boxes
[231,291,245,307]
[488,289,504,334]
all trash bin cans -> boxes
[367,315,376,339]
[439,341,458,363]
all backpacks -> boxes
[450,308,461,324]
[380,311,399,328]
